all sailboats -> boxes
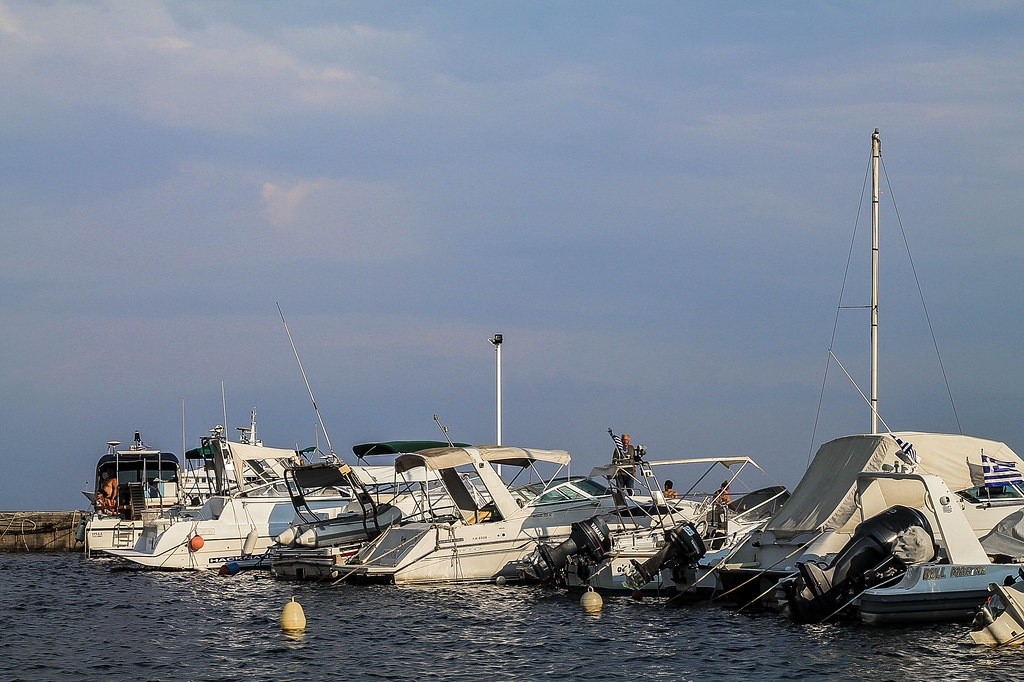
[716,129,1024,628]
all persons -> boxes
[713,480,731,505]
[612,434,635,496]
[94,488,119,517]
[101,468,119,503]
[661,479,677,499]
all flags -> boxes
[981,454,1022,488]
[889,434,917,463]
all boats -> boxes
[103,379,466,572]
[859,564,1024,627]
[562,456,764,596]
[81,429,185,560]
[269,440,533,582]
[82,466,280,507]
[0,511,93,554]
[330,445,618,585]
[672,516,772,594]
